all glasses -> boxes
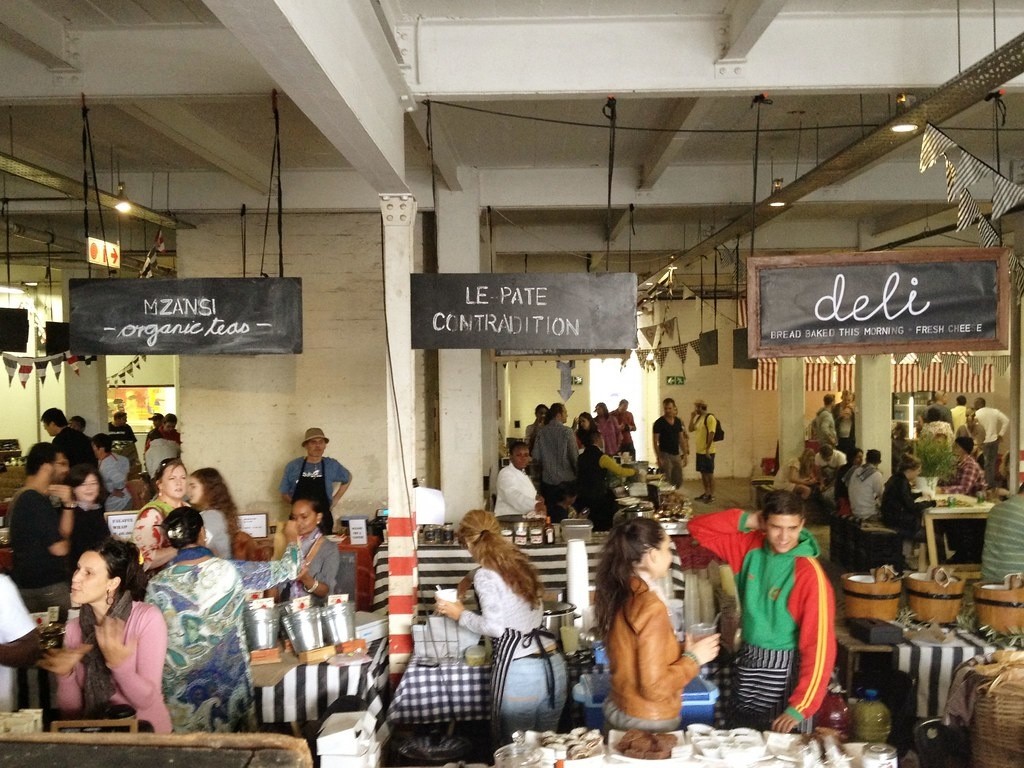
[156,457,174,479]
[55,460,69,468]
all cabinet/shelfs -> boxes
[892,391,936,442]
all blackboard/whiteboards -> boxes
[67,276,303,355]
[746,247,1011,358]
[409,272,640,351]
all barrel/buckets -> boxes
[902,571,966,629]
[972,572,1024,636]
[243,599,356,655]
[840,571,902,622]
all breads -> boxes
[618,728,678,760]
[541,727,604,759]
[801,727,845,759]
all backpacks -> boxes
[704,413,724,442]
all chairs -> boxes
[913,715,972,768]
[872,667,917,758]
[50,719,138,735]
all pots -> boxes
[622,503,655,521]
[539,600,581,640]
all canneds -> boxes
[422,522,454,545]
[501,529,543,546]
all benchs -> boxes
[751,474,775,510]
[826,513,903,579]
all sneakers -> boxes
[706,496,718,504]
[695,493,707,501]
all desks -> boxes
[562,741,871,768]
[253,534,278,603]
[371,530,686,625]
[336,533,381,611]
[251,634,390,737]
[833,623,1024,720]
[916,493,996,580]
[386,646,610,738]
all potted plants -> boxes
[909,429,963,500]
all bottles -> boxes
[501,528,543,546]
[812,683,852,742]
[542,516,555,545]
[850,689,891,744]
[417,522,456,545]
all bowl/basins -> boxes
[561,518,594,541]
[685,721,766,762]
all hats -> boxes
[302,428,329,447]
[149,413,164,419]
[955,437,974,454]
[867,449,881,463]
[694,399,708,407]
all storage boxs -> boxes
[320,741,382,768]
[340,515,369,527]
[561,519,594,542]
[849,618,903,645]
[314,711,378,757]
[249,647,283,666]
[570,672,721,745]
[284,639,294,653]
[762,458,778,476]
[355,610,389,643]
[335,639,366,654]
[297,645,336,664]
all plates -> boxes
[762,730,802,764]
[608,728,693,763]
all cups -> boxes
[689,622,717,642]
[493,741,544,768]
[561,625,579,652]
[566,538,590,610]
[434,588,458,604]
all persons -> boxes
[37,404,243,587]
[982,480,1024,582]
[810,390,856,454]
[281,427,352,536]
[686,490,837,733]
[438,509,569,744]
[892,392,1008,497]
[144,506,305,738]
[494,440,551,518]
[0,569,43,712]
[523,403,639,531]
[653,396,719,504]
[33,538,176,735]
[593,514,721,731]
[771,441,947,568]
[5,441,76,613]
[277,493,340,607]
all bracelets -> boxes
[682,651,697,665]
